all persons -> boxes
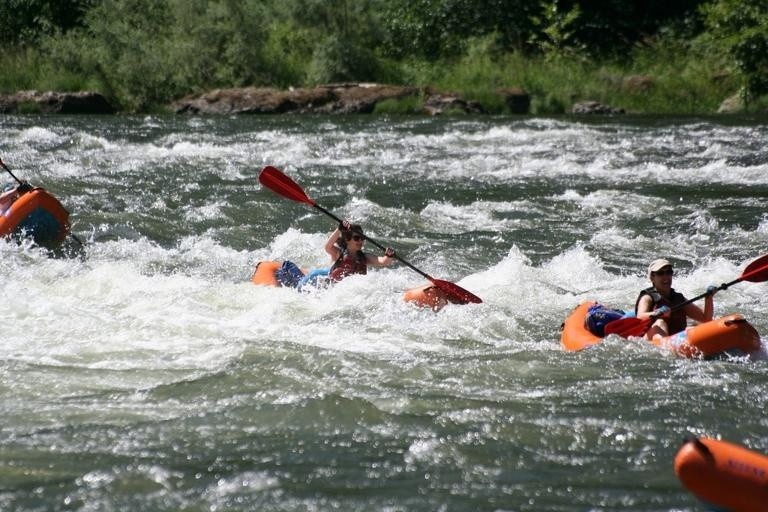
[0,178,30,216]
[634,258,716,343]
[324,220,394,289]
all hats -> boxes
[649,258,673,278]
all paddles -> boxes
[258,167,481,306]
[603,255,768,339]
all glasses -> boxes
[655,269,673,276]
[351,234,367,242]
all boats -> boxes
[563,301,762,360]
[674,428,768,512]
[248,260,483,313]
[0,182,70,254]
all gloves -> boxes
[654,306,671,315]
[17,181,32,194]
[385,248,395,258]
[337,220,351,232]
[705,285,716,297]
